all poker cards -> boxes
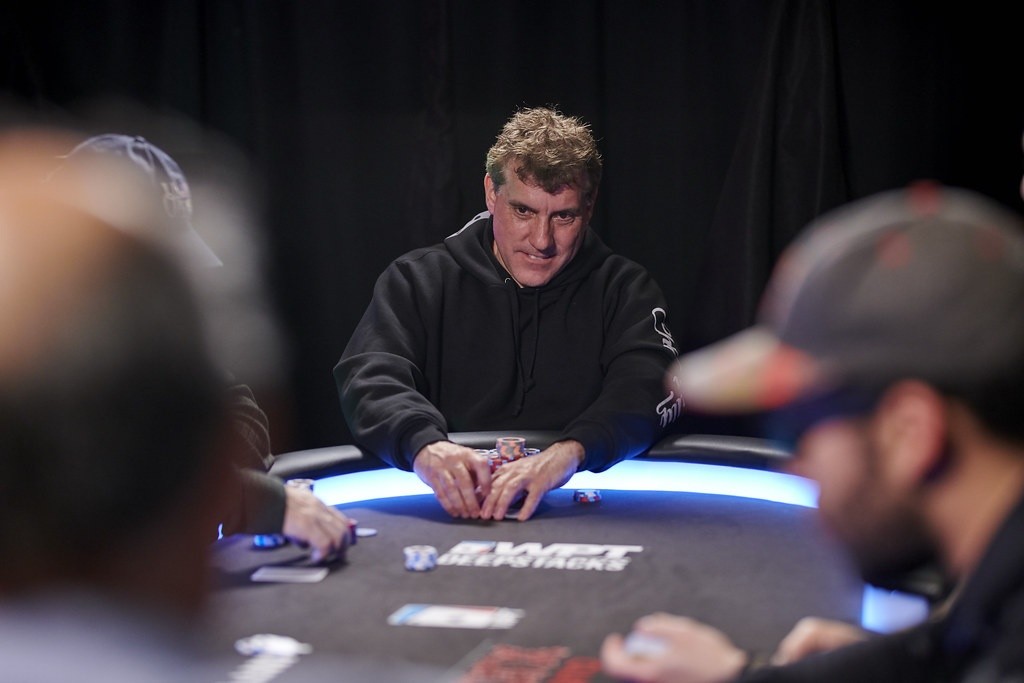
[387,599,520,630]
[251,568,329,585]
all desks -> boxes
[215,432,928,683]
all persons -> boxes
[39,135,358,563]
[0,203,250,682]
[599,187,1024,683]
[333,108,689,521]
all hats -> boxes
[665,185,1024,415]
[66,134,221,268]
